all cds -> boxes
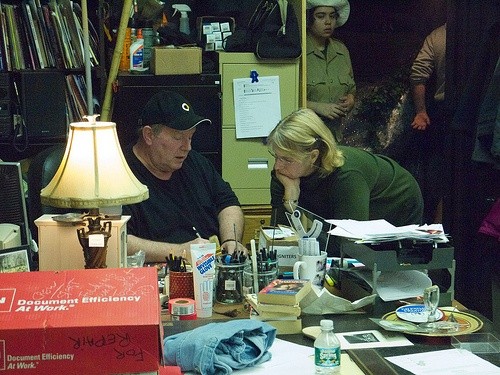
[379,320,418,331]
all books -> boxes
[245,279,312,334]
[0,0,100,143]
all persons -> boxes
[266,108,424,227]
[123,90,249,263]
[306,0,357,126]
[409,23,446,226]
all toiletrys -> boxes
[123,19,154,70]
[190,243,216,318]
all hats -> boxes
[138,90,213,131]
[306,0,350,28]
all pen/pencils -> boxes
[163,253,187,273]
[219,247,246,301]
[193,227,200,239]
[248,248,278,292]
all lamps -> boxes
[41,115,150,270]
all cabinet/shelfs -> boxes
[215,49,300,205]
[114,72,222,175]
[339,235,455,314]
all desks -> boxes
[145,263,500,375]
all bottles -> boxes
[129,29,143,71]
[313,319,342,375]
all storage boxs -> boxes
[196,16,236,51]
[0,267,181,375]
[259,224,300,272]
[150,47,203,75]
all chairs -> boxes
[29,146,89,272]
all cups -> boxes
[212,258,248,305]
[243,258,278,315]
[168,265,194,300]
[293,251,327,287]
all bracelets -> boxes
[282,198,298,204]
[220,239,241,247]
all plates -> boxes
[381,308,483,336]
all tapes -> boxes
[167,297,195,316]
[173,313,198,320]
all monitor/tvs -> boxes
[0,162,30,247]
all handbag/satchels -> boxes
[245,0,301,59]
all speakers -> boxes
[12,71,66,141]
